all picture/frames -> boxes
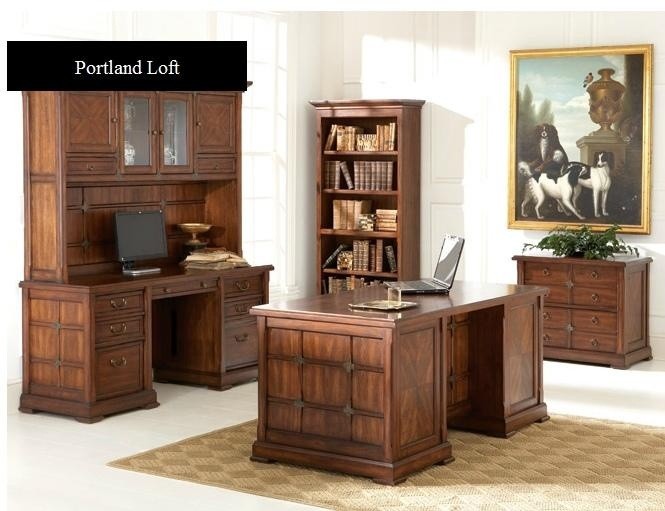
[506,43,654,235]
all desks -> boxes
[248,279,551,487]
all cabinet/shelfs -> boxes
[153,264,275,391]
[193,91,240,178]
[24,92,116,177]
[308,98,426,296]
[19,282,160,424]
[118,92,194,180]
[512,255,654,370]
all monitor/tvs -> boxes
[113,209,168,276]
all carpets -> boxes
[104,412,665,511]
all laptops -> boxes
[382,235,464,295]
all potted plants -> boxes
[521,221,641,259]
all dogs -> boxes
[557,150,614,216]
[517,160,589,220]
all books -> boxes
[321,123,399,293]
[183,247,251,271]
